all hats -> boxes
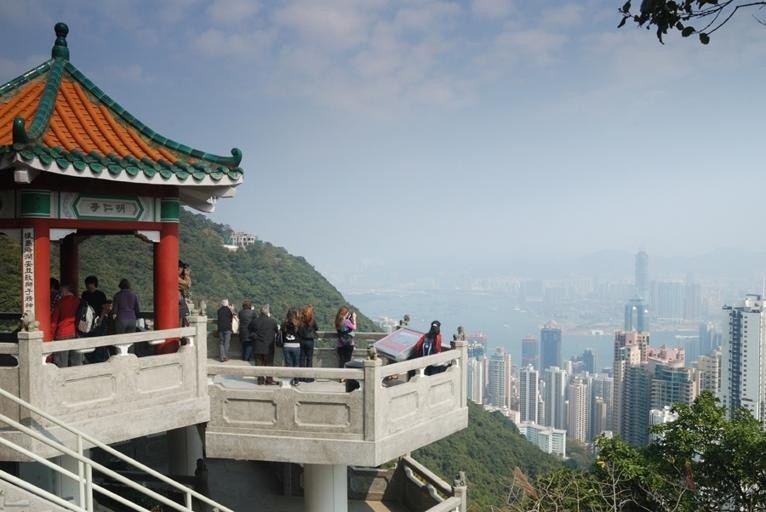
[179,260,188,267]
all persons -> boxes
[336,305,357,367]
[300,304,318,382]
[217,299,234,362]
[186,458,209,512]
[248,304,278,385]
[238,300,257,361]
[50,276,140,368]
[280,307,307,386]
[178,260,191,327]
[416,321,443,375]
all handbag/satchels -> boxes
[342,332,355,343]
[276,331,283,347]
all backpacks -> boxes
[77,301,94,333]
[232,315,239,335]
[422,334,435,357]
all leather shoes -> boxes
[257,377,280,385]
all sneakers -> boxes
[294,379,315,386]
[220,357,229,362]
